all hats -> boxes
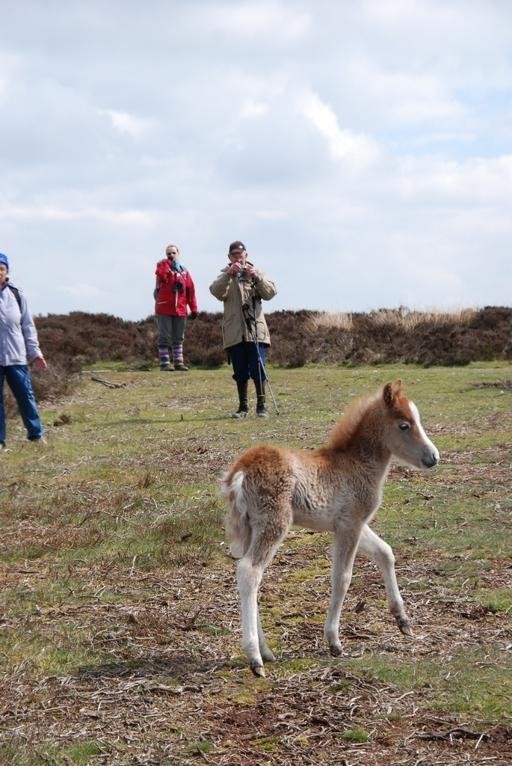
[229,241,244,254]
[0,253,8,264]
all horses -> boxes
[215,379,441,679]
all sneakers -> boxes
[159,365,174,370]
[176,365,188,370]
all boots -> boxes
[232,379,248,417]
[255,378,266,416]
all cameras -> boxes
[240,265,249,272]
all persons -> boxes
[210,240,279,418]
[154,244,198,370]
[0,252,48,448]
